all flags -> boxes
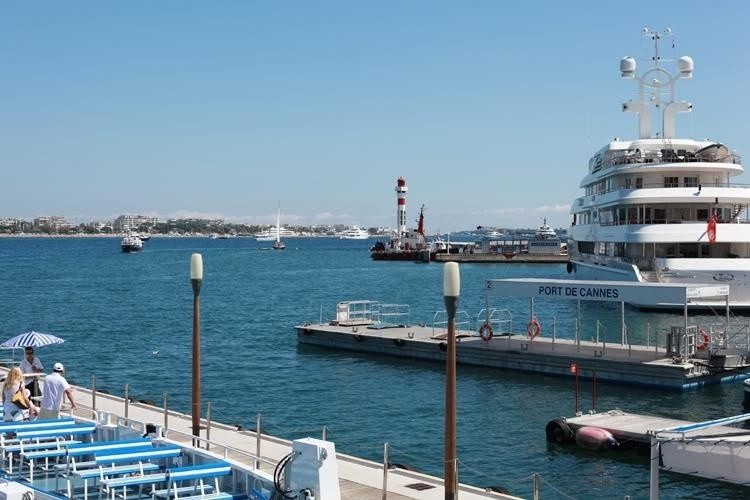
[707,211,716,244]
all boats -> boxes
[120,229,150,250]
[253,233,276,242]
[0,345,541,499]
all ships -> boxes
[339,225,369,240]
[566,24,750,309]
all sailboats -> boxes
[271,208,286,249]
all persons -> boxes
[39,362,77,419]
[19,347,44,407]
[2,366,36,449]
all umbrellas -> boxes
[1,329,68,348]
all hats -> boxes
[53,362,64,371]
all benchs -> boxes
[0,404,252,500]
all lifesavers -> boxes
[479,323,493,341]
[691,329,709,351]
[527,320,540,338]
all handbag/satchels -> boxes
[11,381,29,410]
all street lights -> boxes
[189,252,206,447]
[443,261,461,500]
